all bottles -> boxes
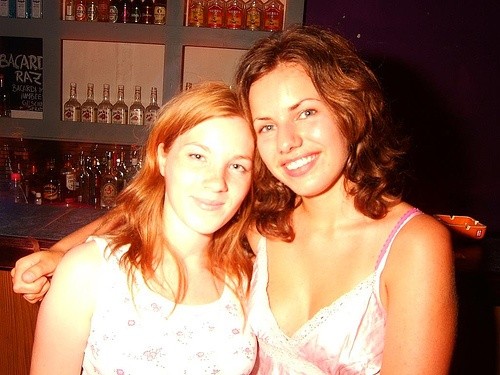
[186,81,192,90]
[144,87,161,125]
[113,85,128,125]
[0,142,146,214]
[29,0,42,19]
[80,83,98,123]
[63,81,81,121]
[15,0,27,19]
[189,0,284,33]
[128,85,145,125]
[98,83,112,124]
[65,0,167,25]
[0,0,10,17]
[0,79,11,117]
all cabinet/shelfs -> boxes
[1,18,303,248]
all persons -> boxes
[10,24,459,375]
[28,79,294,375]
